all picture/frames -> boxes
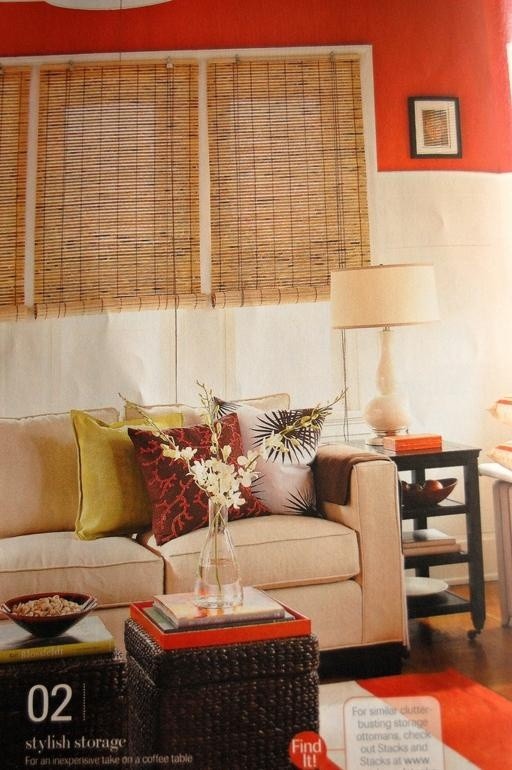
[407,94,463,159]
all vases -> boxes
[191,496,243,608]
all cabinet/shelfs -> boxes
[351,438,490,642]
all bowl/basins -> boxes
[0,592,99,638]
[403,478,458,507]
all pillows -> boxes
[71,395,329,548]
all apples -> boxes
[401,480,443,491]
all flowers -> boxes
[117,380,351,595]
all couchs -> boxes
[0,392,413,683]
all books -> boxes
[144,604,293,633]
[404,544,461,559]
[154,586,284,625]
[403,529,456,548]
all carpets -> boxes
[316,666,512,770]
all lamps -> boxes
[331,260,444,447]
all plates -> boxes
[405,576,449,597]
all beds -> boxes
[123,618,322,769]
